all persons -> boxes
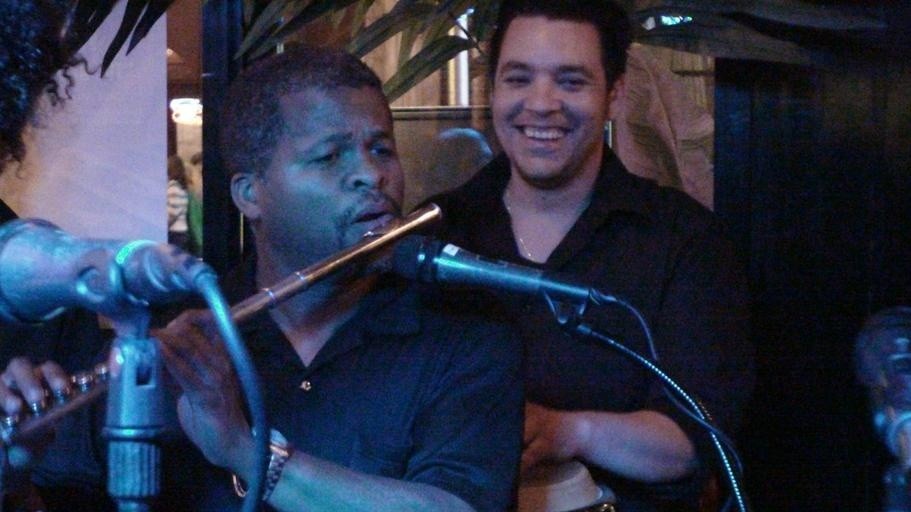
[0,42,527,511]
[409,0,759,511]
[167,155,192,252]
[851,304,911,512]
[0,1,102,364]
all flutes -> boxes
[0,204,442,445]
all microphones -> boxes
[0,218,217,325]
[392,233,622,309]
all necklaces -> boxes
[506,191,536,265]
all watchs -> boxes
[230,423,293,507]
